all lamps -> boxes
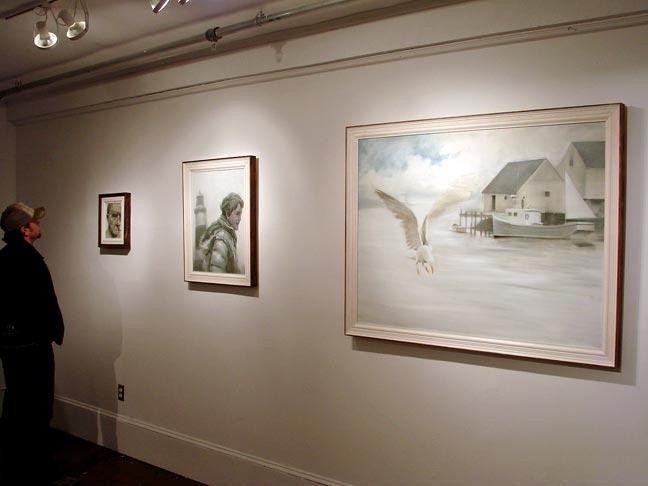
[59,1,89,42]
[33,1,59,49]
[150,0,168,12]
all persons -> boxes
[0,199,65,460]
[102,202,123,240]
[193,192,244,273]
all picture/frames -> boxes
[343,101,626,371]
[182,155,256,289]
[98,192,129,249]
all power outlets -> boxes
[118,384,124,401]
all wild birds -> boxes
[373,187,465,276]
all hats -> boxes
[0,201,46,233]
